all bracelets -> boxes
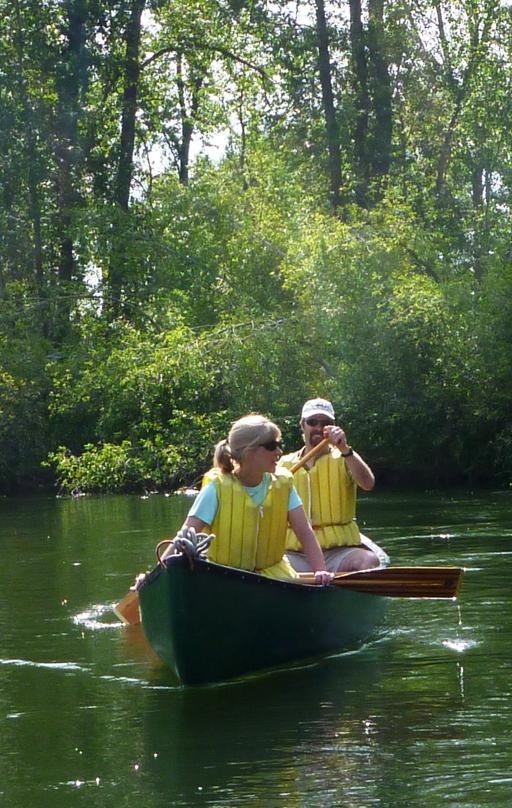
[340,447,353,457]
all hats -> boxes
[301,397,336,422]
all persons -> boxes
[266,396,383,571]
[129,413,332,591]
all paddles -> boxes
[114,432,341,625]
[293,567,462,598]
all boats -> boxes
[133,531,394,690]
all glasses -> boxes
[306,419,333,427]
[235,438,286,451]
